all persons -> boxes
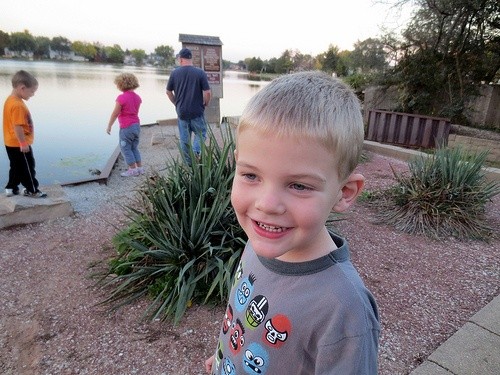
[3,69,47,199]
[205,69,380,375]
[105,72,145,177]
[166,48,210,178]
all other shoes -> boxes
[121,169,139,177]
[26,190,47,198]
[194,154,201,161]
[137,168,145,175]
[5,187,19,196]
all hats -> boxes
[176,48,193,60]
